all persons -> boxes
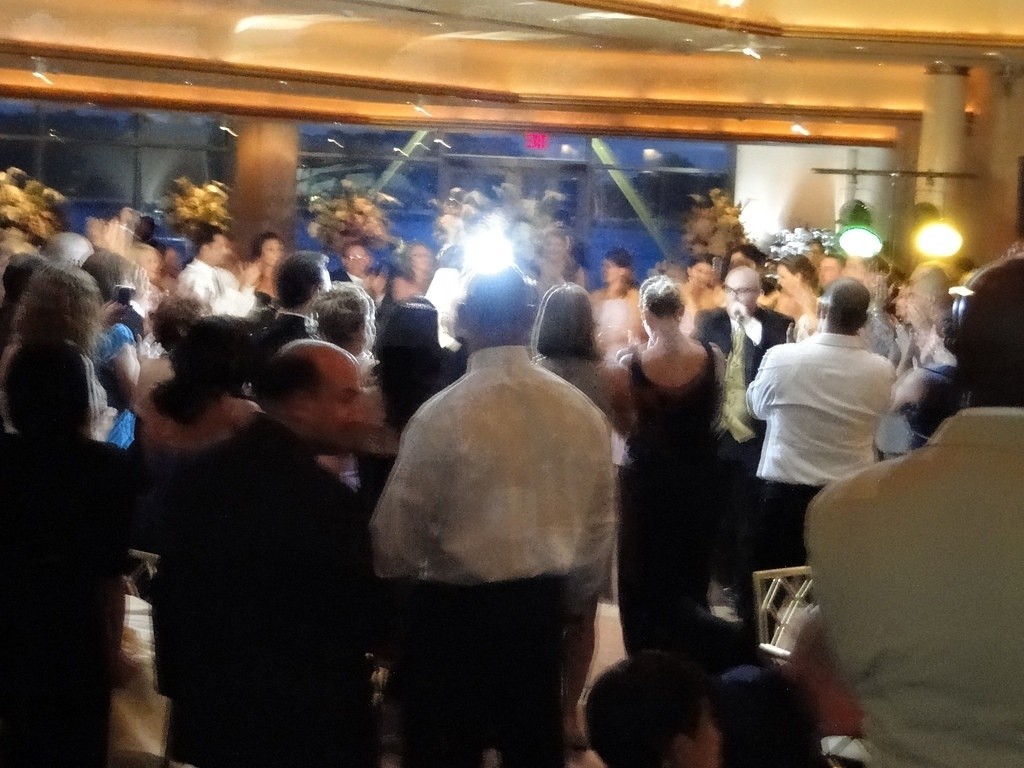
[0,166,1024,768]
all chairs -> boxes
[751,567,868,768]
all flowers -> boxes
[0,168,62,242]
[162,175,235,235]
[304,177,408,254]
[429,179,571,282]
[683,189,747,263]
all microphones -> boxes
[734,311,744,324]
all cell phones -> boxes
[112,284,130,306]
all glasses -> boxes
[722,285,758,295]
[344,254,365,261]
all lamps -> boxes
[911,203,962,259]
[836,202,881,257]
